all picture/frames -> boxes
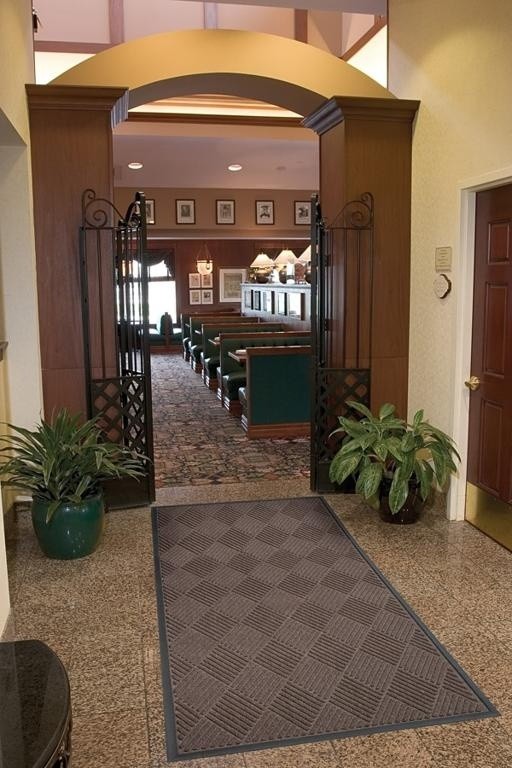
[216,199,235,225]
[244,290,305,321]
[216,266,248,305]
[255,200,275,225]
[294,200,312,226]
[135,199,155,225]
[175,199,196,224]
[188,272,214,305]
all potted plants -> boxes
[0,407,154,559]
[327,400,461,525]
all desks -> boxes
[0,640,72,768]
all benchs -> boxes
[179,310,313,438]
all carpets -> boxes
[151,496,501,763]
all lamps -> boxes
[250,245,319,285]
[194,241,214,275]
